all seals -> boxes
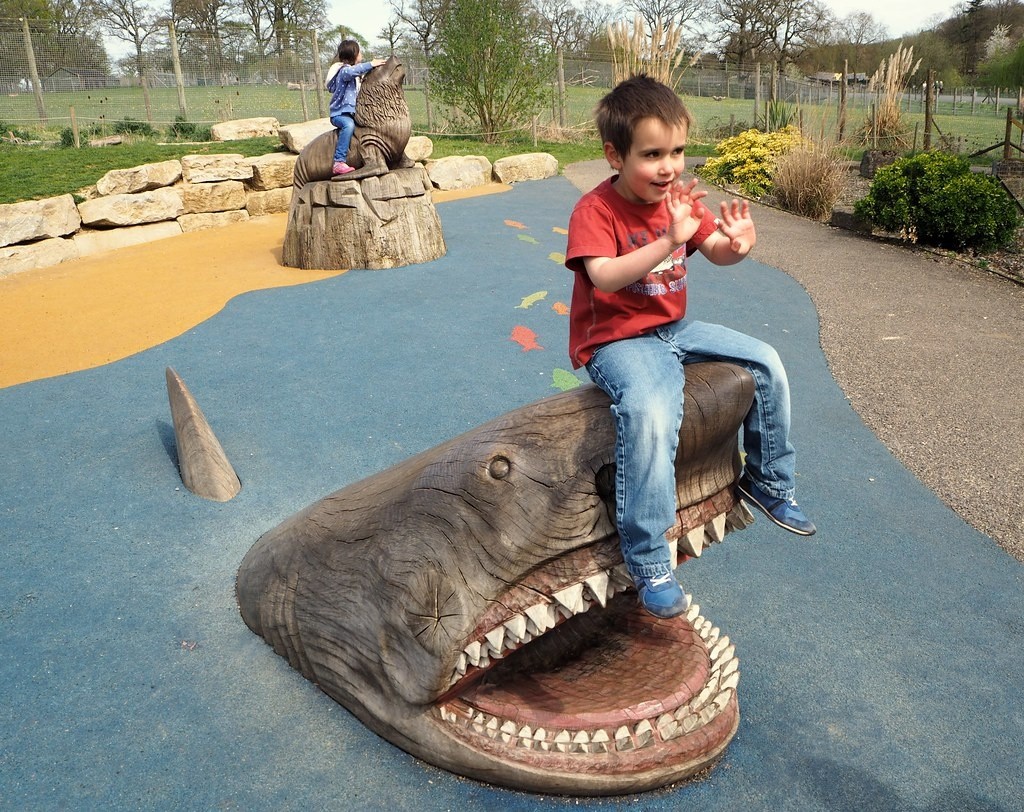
[285,54,416,229]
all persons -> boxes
[325,39,387,174]
[563,71,817,620]
